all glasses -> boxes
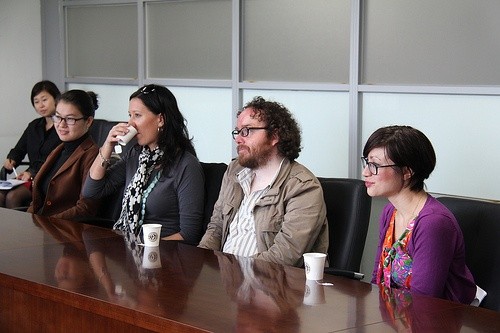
[232,127,274,140]
[137,86,155,95]
[51,113,89,125]
[361,157,397,175]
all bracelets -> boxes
[99,149,110,167]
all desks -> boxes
[0,208,500,333]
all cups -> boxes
[116,124,138,145]
[141,223,162,247]
[141,246,161,270]
[303,279,326,306]
[303,252,327,280]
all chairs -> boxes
[313,177,373,281]
[0,119,141,225]
[433,196,500,313]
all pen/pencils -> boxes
[13,167,18,178]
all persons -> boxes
[363,126,477,303]
[27,90,100,221]
[197,96,328,268]
[31,213,467,333]
[81,84,203,240]
[0,80,63,210]
